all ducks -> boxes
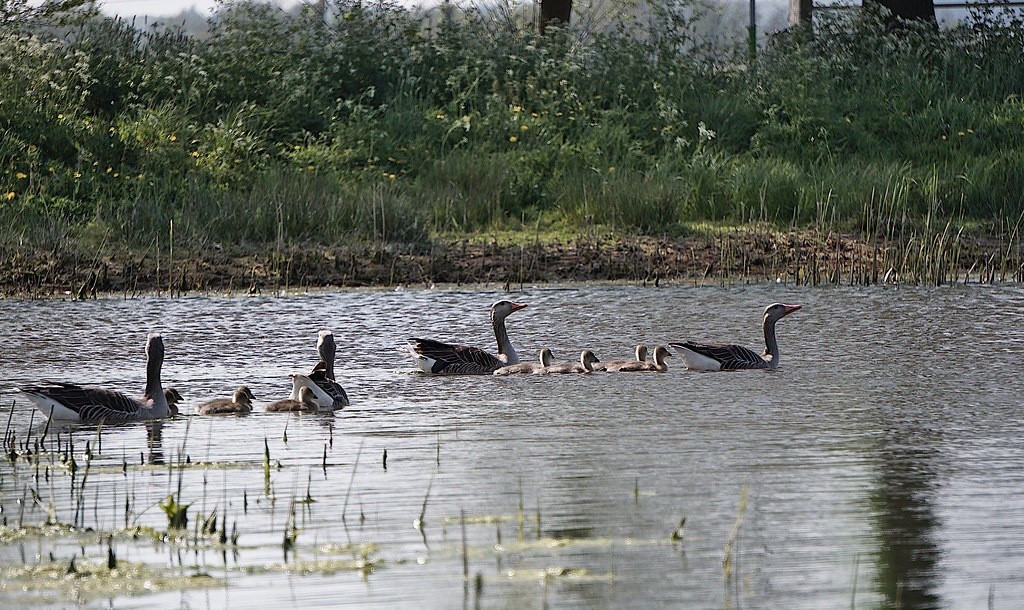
[405,300,805,376]
[15,329,351,423]
